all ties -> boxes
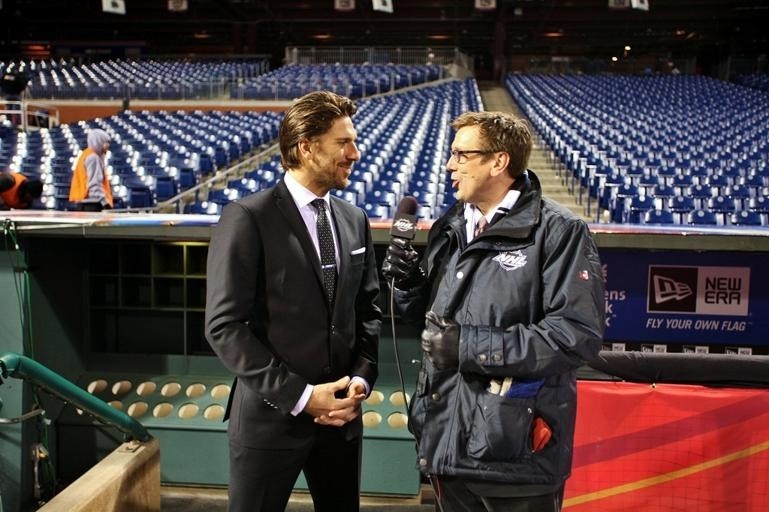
[473,218,487,240]
[310,198,339,314]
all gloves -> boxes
[382,237,420,285]
[420,309,462,373]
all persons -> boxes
[0,170,43,211]
[201,91,383,511]
[68,127,117,212]
[382,110,606,510]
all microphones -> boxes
[389,195,417,281]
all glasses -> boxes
[449,149,498,164]
